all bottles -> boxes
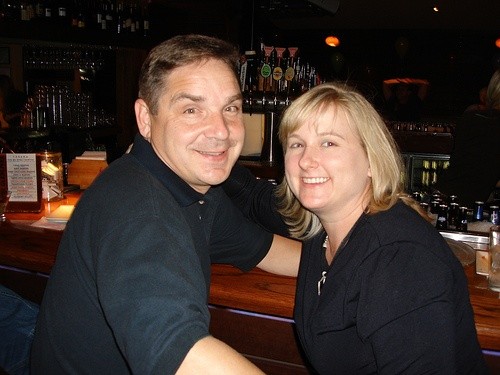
[0,0,151,38]
[23,44,118,70]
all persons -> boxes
[435,69,500,209]
[0,74,26,129]
[463,86,489,114]
[382,85,432,122]
[30,35,302,375]
[219,81,491,375]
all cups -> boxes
[488,225,500,292]
[41,151,64,201]
[412,191,500,231]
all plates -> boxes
[444,236,476,265]
